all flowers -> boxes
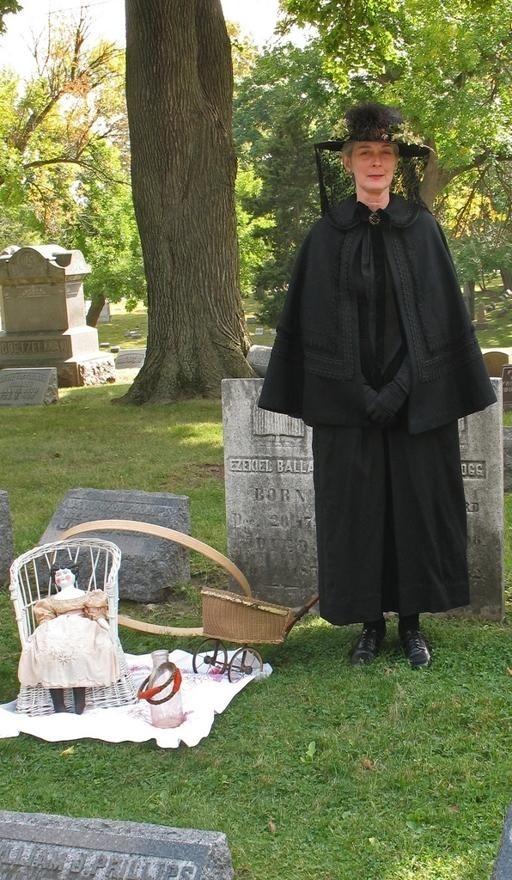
[380,122,425,146]
[329,117,352,142]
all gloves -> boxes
[367,380,408,424]
[354,381,384,421]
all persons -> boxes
[17,557,128,715]
[252,101,500,672]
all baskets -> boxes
[201,586,320,646]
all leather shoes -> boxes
[350,619,387,668]
[397,618,432,668]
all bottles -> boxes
[147,647,185,730]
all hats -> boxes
[314,100,437,159]
